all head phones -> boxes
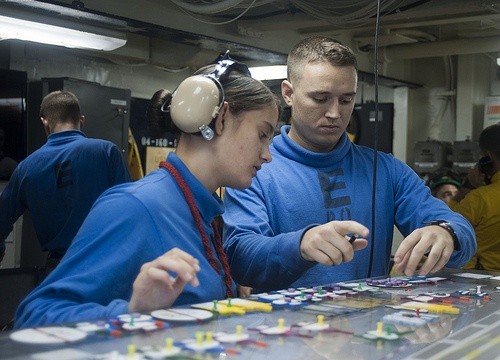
[167,60,251,138]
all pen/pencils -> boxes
[350,233,358,244]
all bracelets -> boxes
[430,221,456,243]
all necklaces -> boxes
[159,162,232,299]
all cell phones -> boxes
[478,155,492,175]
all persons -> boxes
[13,61,278,331]
[222,37,478,295]
[452,122,500,272]
[0,90,131,270]
